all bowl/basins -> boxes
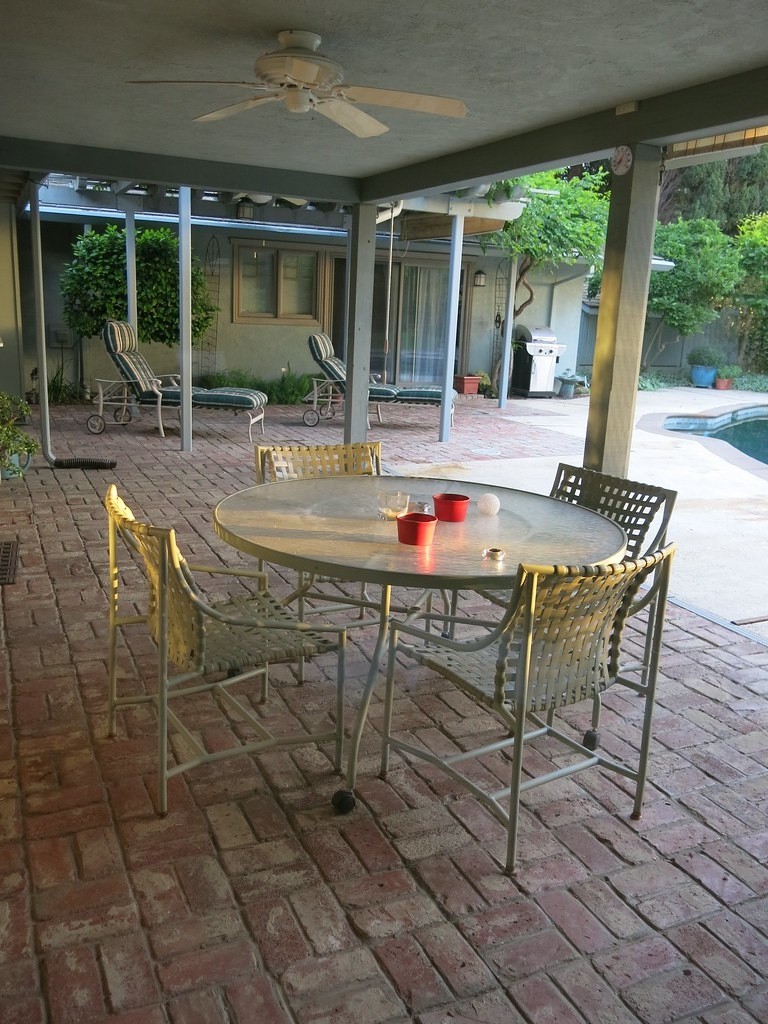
[377,490,410,520]
[433,493,470,521]
[397,513,438,545]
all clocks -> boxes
[610,145,633,176]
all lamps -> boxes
[235,200,254,221]
[473,269,488,287]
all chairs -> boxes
[104,485,347,819]
[449,460,679,751]
[85,319,268,443]
[378,540,676,877]
[254,439,433,651]
[302,333,458,427]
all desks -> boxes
[212,474,628,814]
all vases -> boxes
[454,375,482,395]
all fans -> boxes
[124,30,468,139]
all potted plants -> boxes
[0,392,43,478]
[728,364,743,389]
[686,345,727,389]
[715,367,732,390]
